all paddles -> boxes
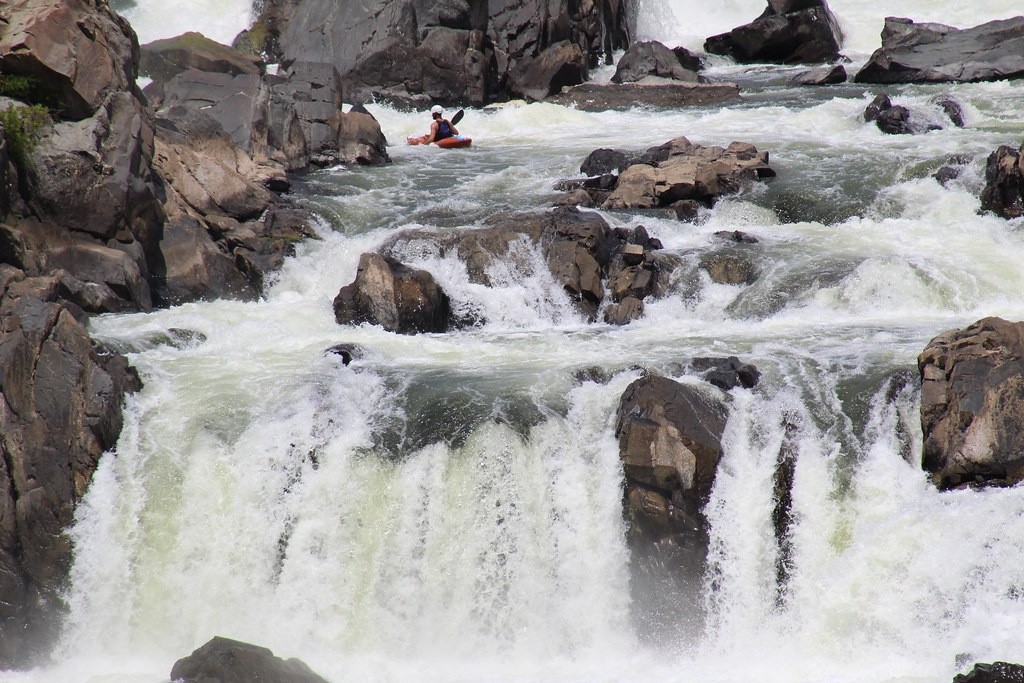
[422,109,464,144]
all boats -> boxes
[408,132,471,149]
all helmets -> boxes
[431,103,443,114]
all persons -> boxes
[423,105,459,145]
